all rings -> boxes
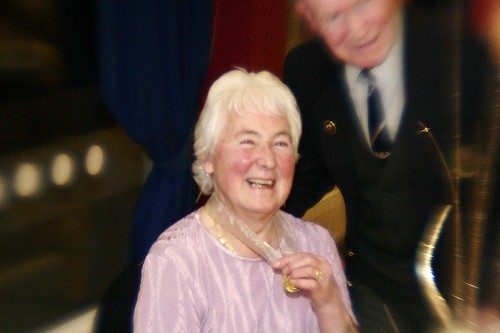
[315,267,323,284]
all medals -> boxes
[282,272,302,293]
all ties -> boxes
[361,68,394,158]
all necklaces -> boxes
[214,220,273,258]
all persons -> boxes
[256,1,500,333]
[132,62,362,332]
